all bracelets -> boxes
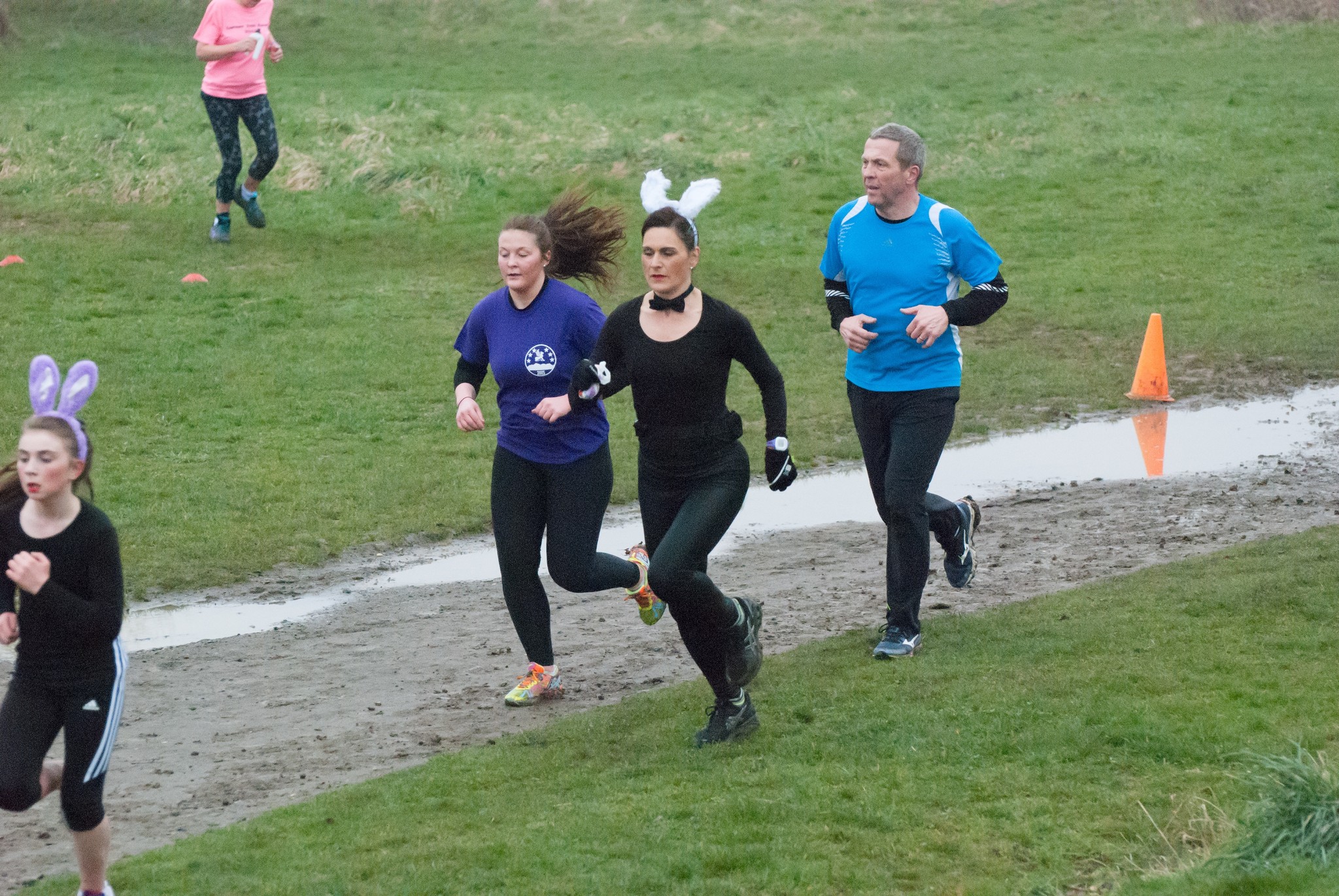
[458,396,475,408]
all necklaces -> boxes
[648,282,694,313]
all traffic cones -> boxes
[1131,409,1169,478]
[1124,312,1176,401]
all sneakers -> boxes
[234,184,266,228]
[713,597,764,685]
[623,544,667,624]
[942,496,981,586]
[209,210,231,244]
[693,690,759,749]
[504,662,560,705]
[873,622,920,660]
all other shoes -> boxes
[77,880,115,896]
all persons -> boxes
[454,190,666,707]
[817,123,1011,659]
[0,355,129,896]
[193,0,285,244]
[569,170,799,752]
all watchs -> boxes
[765,436,790,452]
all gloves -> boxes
[765,447,798,492]
[570,359,601,391]
